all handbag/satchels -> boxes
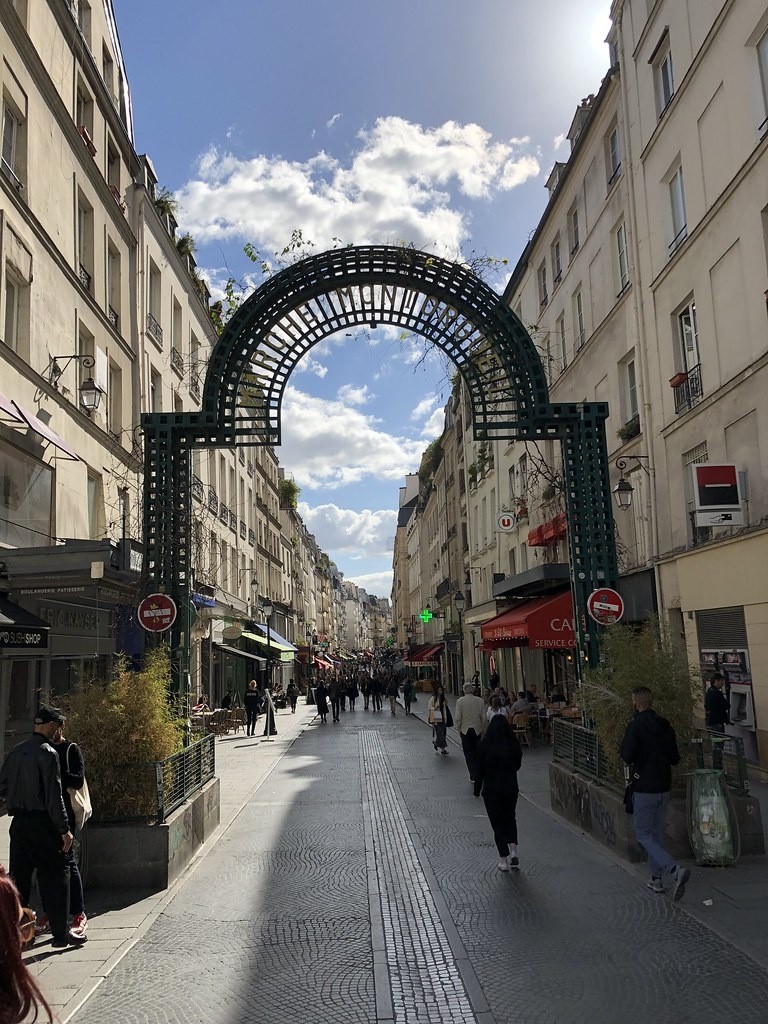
[430,710,442,723]
[623,781,636,814]
[66,742,92,830]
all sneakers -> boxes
[647,875,665,892]
[671,864,690,900]
[69,913,89,935]
[35,914,52,935]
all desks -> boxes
[194,712,214,733]
[217,708,233,728]
[539,713,583,744]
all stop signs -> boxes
[137,592,177,632]
[587,587,624,625]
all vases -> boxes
[669,372,688,388]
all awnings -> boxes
[481,591,576,648]
[0,596,51,648]
[242,624,299,660]
[214,642,267,671]
[317,653,357,669]
[404,644,444,666]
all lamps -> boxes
[612,453,650,512]
[426,602,432,612]
[403,623,408,630]
[464,564,481,591]
[239,567,260,593]
[299,616,303,627]
[47,353,103,416]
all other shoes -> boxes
[469,779,475,783]
[432,741,438,750]
[510,858,518,867]
[441,750,449,754]
[498,862,508,871]
[336,718,340,721]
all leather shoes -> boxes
[52,932,86,947]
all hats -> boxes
[33,707,67,724]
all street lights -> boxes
[454,590,465,698]
[406,628,416,681]
[262,596,277,688]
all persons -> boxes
[244,680,260,735]
[472,671,480,695]
[0,705,86,947]
[34,714,87,935]
[473,714,523,871]
[311,656,424,723]
[222,690,233,710]
[490,669,499,683]
[272,679,298,713]
[456,684,487,782]
[0,863,53,1024]
[428,685,450,754]
[704,673,735,780]
[484,684,566,747]
[619,687,691,900]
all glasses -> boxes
[18,908,37,942]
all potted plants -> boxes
[542,484,555,498]
[615,420,640,443]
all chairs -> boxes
[190,707,246,738]
[509,691,582,749]
